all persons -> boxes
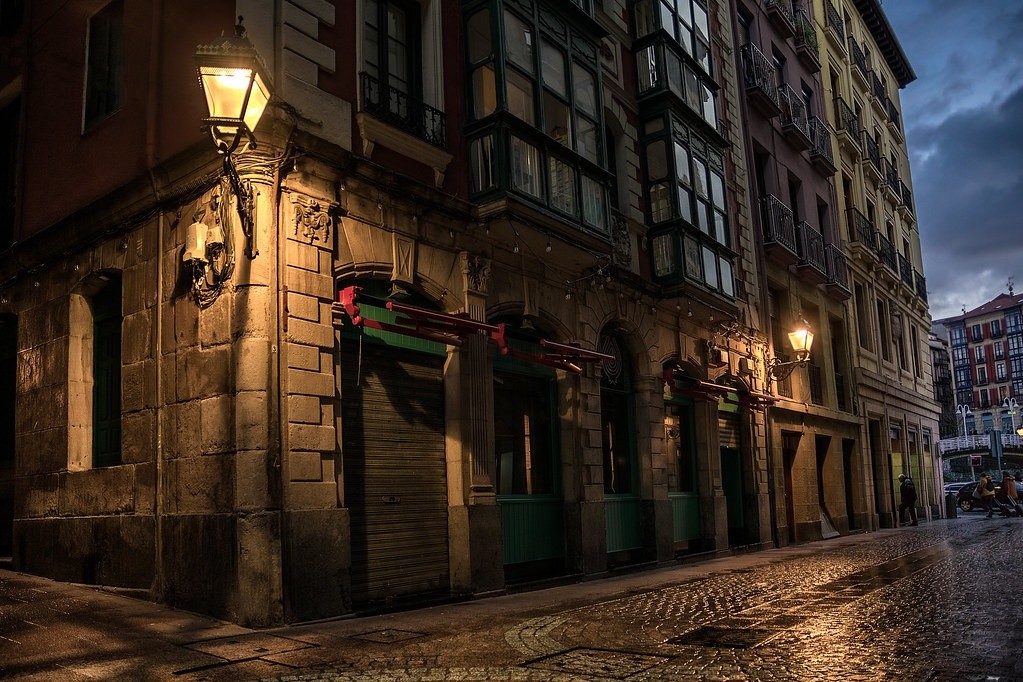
[898,474,917,526]
[1000,471,1017,515]
[979,473,995,517]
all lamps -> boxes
[192,15,276,258]
[770,313,813,381]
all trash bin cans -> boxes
[946,493,957,519]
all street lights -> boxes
[1002,397,1020,445]
[955,404,975,447]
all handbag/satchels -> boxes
[973,488,982,499]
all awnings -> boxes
[671,372,780,405]
[357,291,499,332]
[506,326,615,363]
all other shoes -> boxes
[908,522,919,526]
[985,515,992,517]
[997,512,1007,516]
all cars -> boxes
[944,480,1023,512]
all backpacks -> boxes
[984,478,994,491]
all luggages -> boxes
[994,497,1011,517]
[1007,495,1023,516]
